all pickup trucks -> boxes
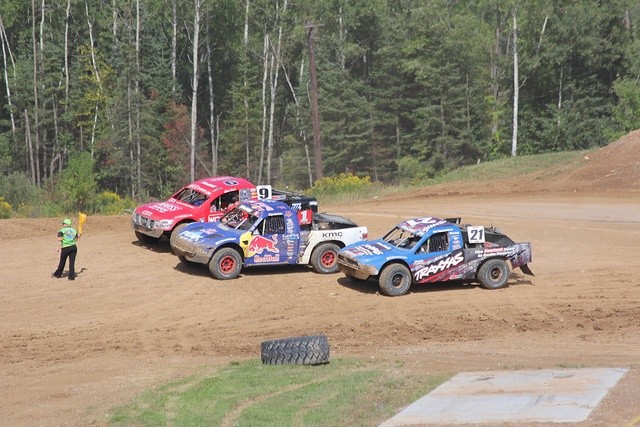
[131,176,318,243]
[336,217,535,296]
[170,199,368,280]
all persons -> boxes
[52,218,81,279]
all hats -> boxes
[63,219,71,225]
